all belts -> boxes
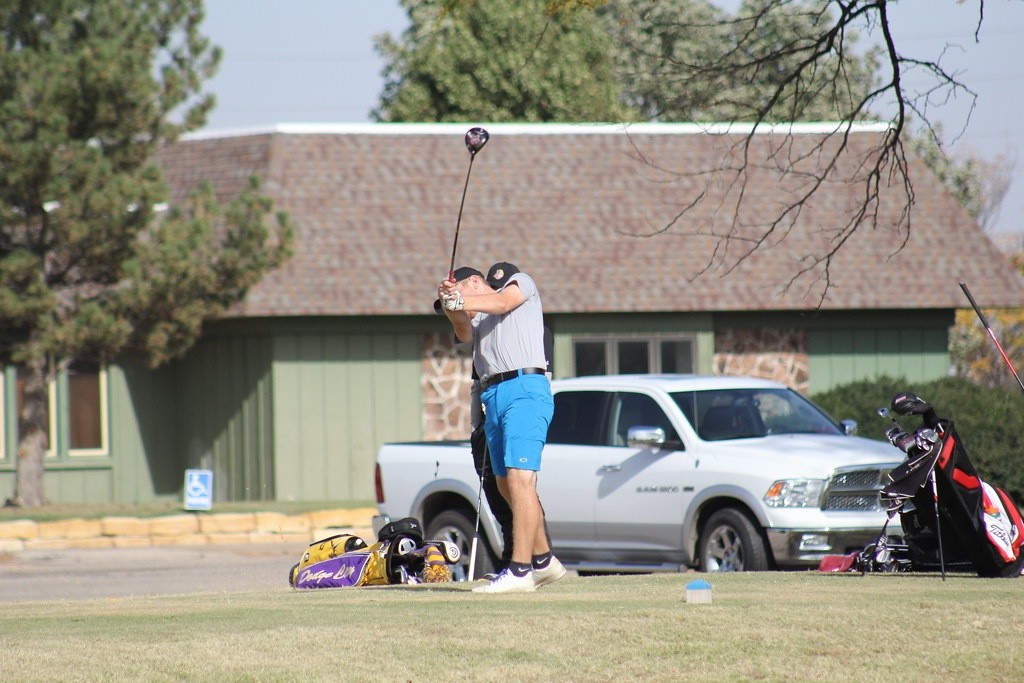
[480,367,545,393]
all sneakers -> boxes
[532,554,566,589]
[471,567,534,593]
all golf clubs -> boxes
[958,279,1024,391]
[467,435,488,581]
[877,392,940,453]
[433,127,490,313]
[392,537,462,585]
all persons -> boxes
[433,266,567,595]
[471,260,554,580]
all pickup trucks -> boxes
[371,372,916,582]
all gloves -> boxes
[441,291,464,312]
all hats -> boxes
[486,262,520,288]
[434,266,484,314]
